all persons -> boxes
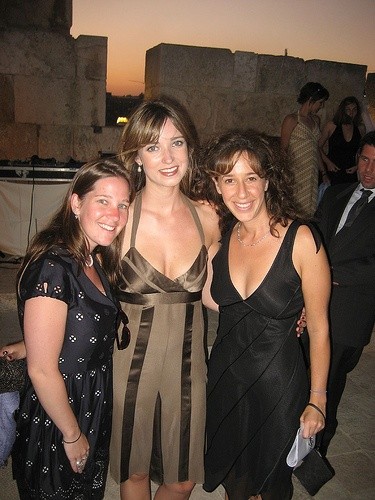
[313,131,375,457]
[280,81,366,217]
[0,98,308,500]
[11,157,132,500]
[0,340,27,361]
[195,128,330,500]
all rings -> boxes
[83,457,87,460]
[77,461,84,471]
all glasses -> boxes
[113,299,130,350]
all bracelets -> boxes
[63,431,81,444]
[310,390,327,393]
[320,170,327,176]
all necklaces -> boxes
[85,254,93,267]
[237,223,270,246]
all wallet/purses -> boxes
[291,448,337,496]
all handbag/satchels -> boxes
[0,351,26,468]
[286,428,319,469]
[317,181,335,205]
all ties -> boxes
[334,187,374,242]
